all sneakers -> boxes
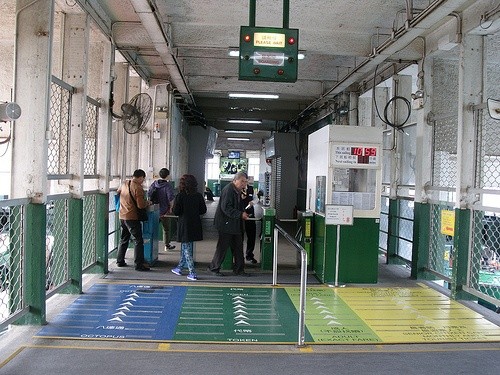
[187,273,198,281]
[164,245,176,252]
[171,268,182,276]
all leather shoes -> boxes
[246,256,257,264]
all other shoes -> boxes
[207,265,224,276]
[233,270,251,277]
[117,261,127,267]
[136,264,150,271]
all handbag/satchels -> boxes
[136,206,149,221]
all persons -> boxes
[148,168,176,250]
[206,186,214,197]
[172,174,207,280]
[239,172,258,264]
[116,169,152,271]
[204,181,214,201]
[209,172,249,277]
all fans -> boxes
[0,101,23,144]
[109,82,153,134]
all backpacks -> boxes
[150,183,159,204]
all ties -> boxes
[243,189,245,197]
